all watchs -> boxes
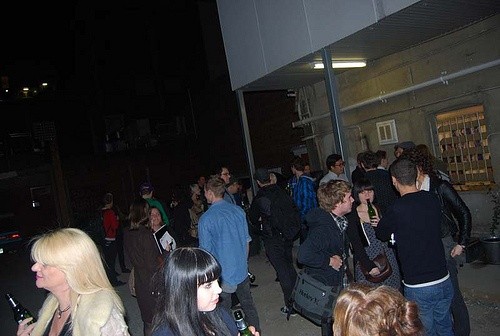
[461,245,466,249]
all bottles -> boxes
[366,199,377,223]
[233,310,255,336]
[4,291,37,330]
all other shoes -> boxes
[111,280,127,287]
[280,306,299,317]
[122,269,131,273]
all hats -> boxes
[140,182,151,191]
[254,168,269,180]
[394,141,416,150]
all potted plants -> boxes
[476,180,500,264]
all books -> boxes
[152,224,173,254]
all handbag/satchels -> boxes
[291,269,339,326]
[128,266,136,297]
[360,253,393,283]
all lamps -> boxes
[314,60,367,69]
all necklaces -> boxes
[58,304,70,319]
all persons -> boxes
[331,283,422,336]
[249,167,307,316]
[99,187,131,286]
[403,145,471,336]
[150,247,260,336]
[174,166,255,308]
[320,154,349,187]
[289,155,324,245]
[352,141,414,216]
[123,188,177,336]
[348,178,404,293]
[376,158,453,336]
[297,180,355,336]
[198,176,262,336]
[17,228,130,336]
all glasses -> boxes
[331,161,346,168]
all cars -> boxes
[0,227,23,248]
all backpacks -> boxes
[262,189,302,240]
[89,208,114,239]
